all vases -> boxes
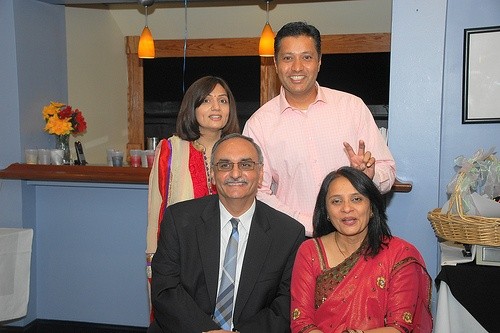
[57,134,70,164]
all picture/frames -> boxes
[462,26,500,124]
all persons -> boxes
[151,133,305,333]
[145,76,241,286]
[290,165,433,333]
[242,22,396,239]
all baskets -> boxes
[425,162,499,247]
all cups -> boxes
[106,138,158,169]
[24,147,63,165]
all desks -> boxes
[0,160,152,328]
[433,261,500,333]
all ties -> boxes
[211,218,241,330]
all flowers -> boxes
[41,100,88,137]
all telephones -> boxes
[74,141,87,165]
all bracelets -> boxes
[346,327,364,333]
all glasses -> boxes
[214,159,259,171]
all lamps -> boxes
[136,0,156,59]
[256,0,276,58]
